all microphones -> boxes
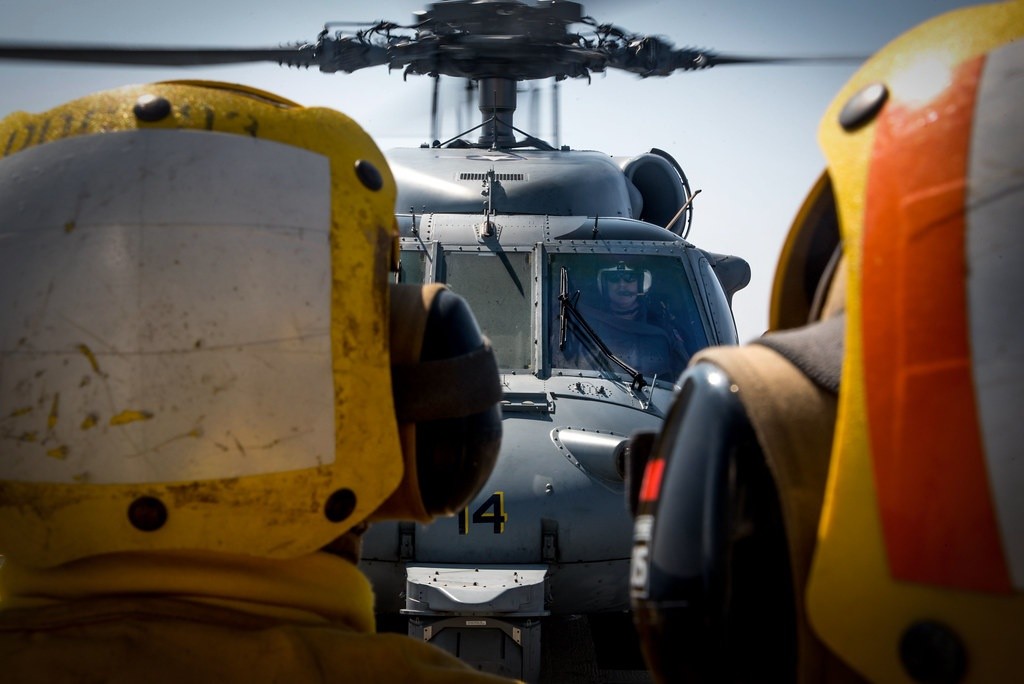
[620,290,645,299]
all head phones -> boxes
[597,266,653,299]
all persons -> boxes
[555,260,688,384]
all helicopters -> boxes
[0,0,892,571]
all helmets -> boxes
[596,254,648,296]
[0,78,406,560]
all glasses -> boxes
[605,270,639,284]
